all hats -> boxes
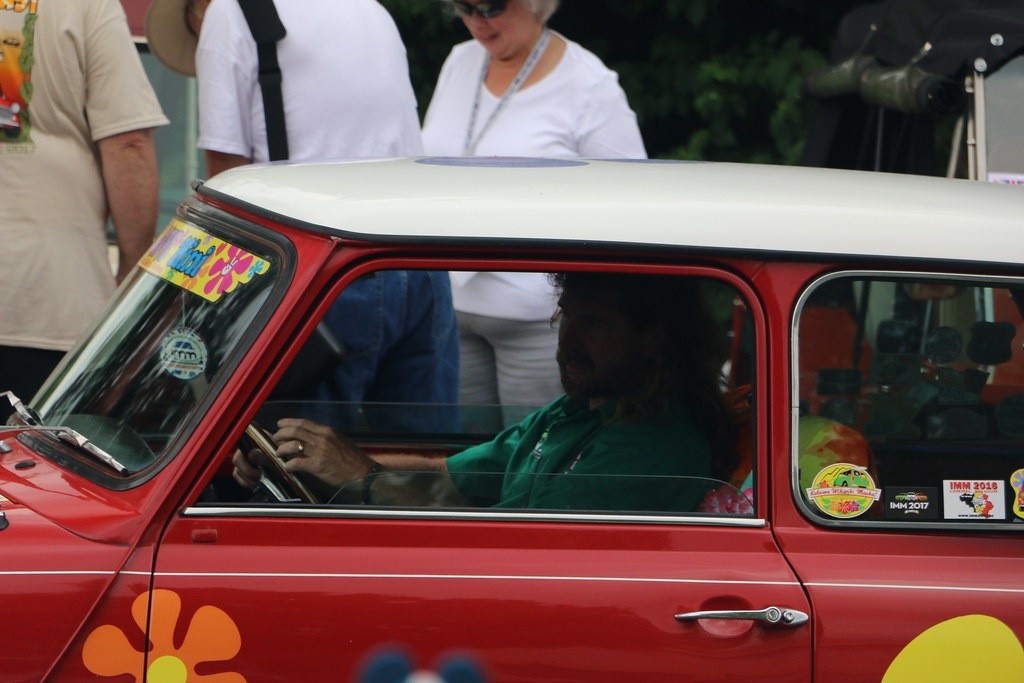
[144,0,200,77]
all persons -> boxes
[0,0,172,436]
[232,271,728,516]
[421,0,648,435]
[183,0,462,435]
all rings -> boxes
[298,439,304,455]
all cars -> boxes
[0,154,1024,683]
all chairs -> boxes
[705,416,881,517]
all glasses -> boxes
[453,0,510,18]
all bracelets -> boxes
[361,461,384,505]
